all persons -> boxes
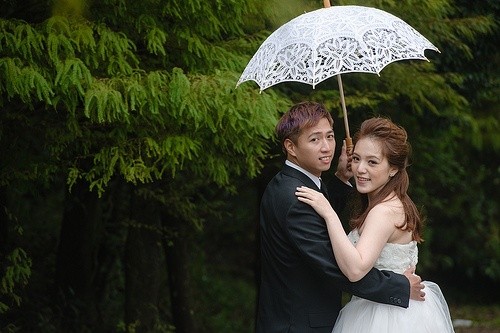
[256,102,426,333]
[294,117,455,333]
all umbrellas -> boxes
[234,0,441,158]
